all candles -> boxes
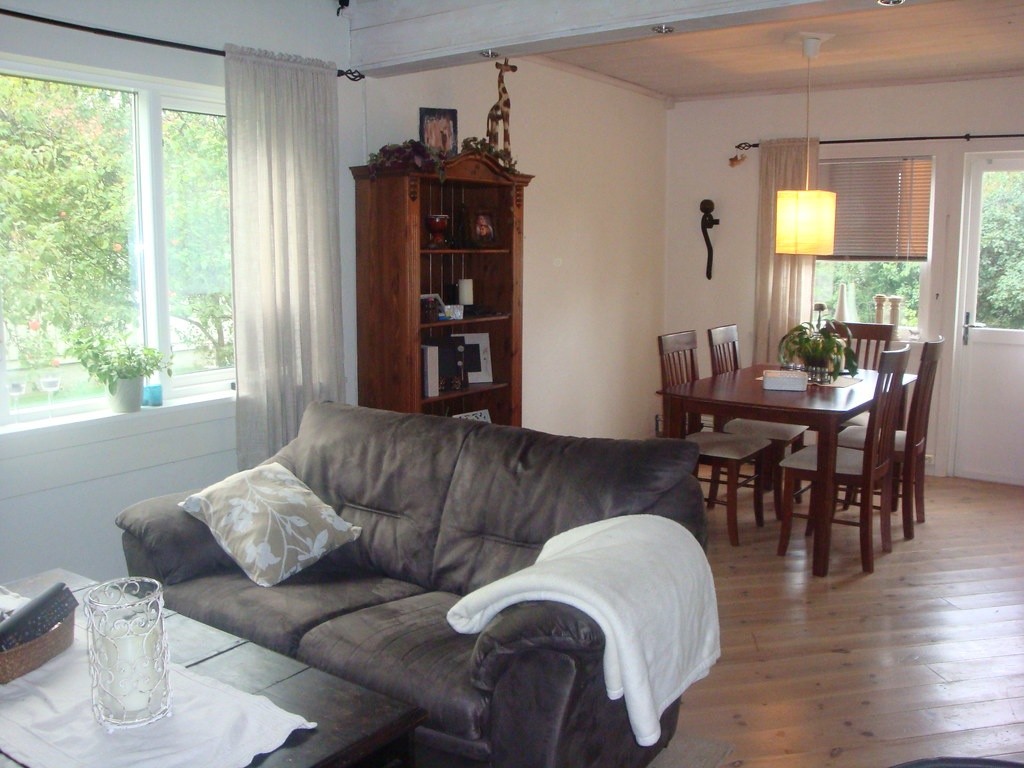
[459,278,473,305]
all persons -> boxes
[476,213,494,245]
[439,130,448,157]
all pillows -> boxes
[178,458,363,590]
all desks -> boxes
[655,362,919,578]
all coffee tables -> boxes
[0,567,431,768]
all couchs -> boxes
[106,397,721,768]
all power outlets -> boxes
[925,455,934,465]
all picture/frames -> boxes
[421,332,494,398]
[420,106,458,156]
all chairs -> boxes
[658,322,946,574]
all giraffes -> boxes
[486,55,518,169]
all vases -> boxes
[85,574,172,730]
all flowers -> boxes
[362,134,514,200]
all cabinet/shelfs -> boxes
[348,147,535,427]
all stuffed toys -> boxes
[486,57,521,175]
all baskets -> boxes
[0,585,75,684]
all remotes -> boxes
[0,580,79,653]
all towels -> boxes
[445,513,722,746]
[0,584,320,768]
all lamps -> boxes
[774,32,837,256]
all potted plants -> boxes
[76,327,175,414]
[776,318,859,384]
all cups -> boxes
[83,576,174,731]
[421,299,438,323]
